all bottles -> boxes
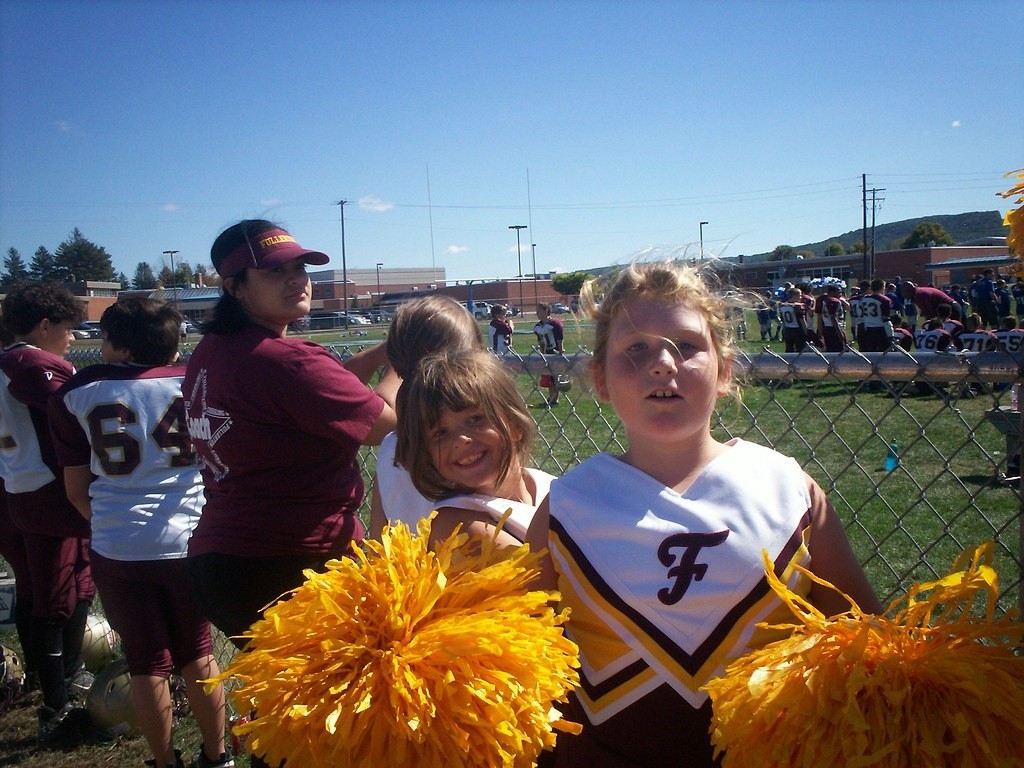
[1011,380,1021,411]
[885,438,898,471]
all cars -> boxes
[459,302,520,320]
[550,302,573,315]
[68,320,102,339]
[287,308,391,326]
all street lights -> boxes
[532,243,537,305]
[699,222,708,263]
[508,225,529,317]
[376,262,384,325]
[163,250,182,310]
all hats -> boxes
[220,229,329,281]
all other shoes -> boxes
[191,742,235,768]
[145,748,184,768]
[539,399,560,407]
[65,661,97,701]
[38,700,75,740]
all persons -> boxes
[181,220,404,768]
[532,301,565,407]
[528,254,883,768]
[487,303,514,355]
[0,277,560,768]
[722,267,1024,399]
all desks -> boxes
[985,405,1024,488]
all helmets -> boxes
[556,374,573,392]
[0,644,24,711]
[86,658,143,738]
[79,613,119,667]
